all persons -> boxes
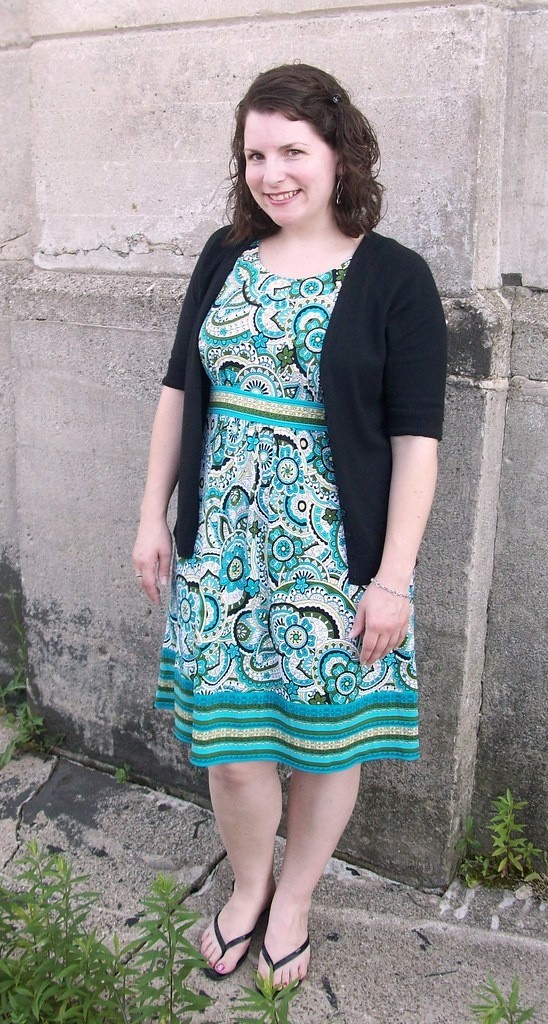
[133,65,447,999]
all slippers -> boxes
[256,935,310,1000]
[198,911,254,981]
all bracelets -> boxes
[371,579,412,599]
